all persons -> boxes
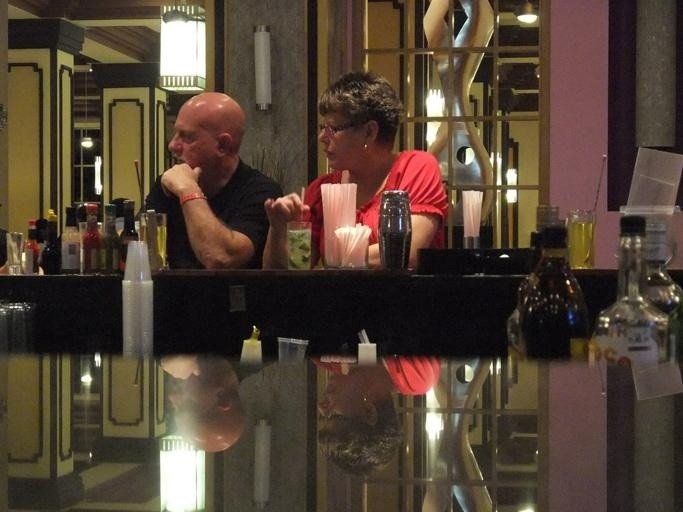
[134,92,282,269]
[157,355,272,452]
[309,355,441,479]
[260,71,449,271]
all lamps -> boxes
[158,0,209,94]
[77,67,95,150]
[158,435,205,512]
[254,23,275,111]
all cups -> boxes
[0,302,32,353]
[5,231,22,275]
[118,240,153,359]
[276,336,309,368]
[321,223,369,271]
[286,222,312,270]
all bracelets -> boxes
[176,193,210,202]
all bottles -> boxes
[377,190,411,273]
[515,203,682,365]
[22,198,168,276]
